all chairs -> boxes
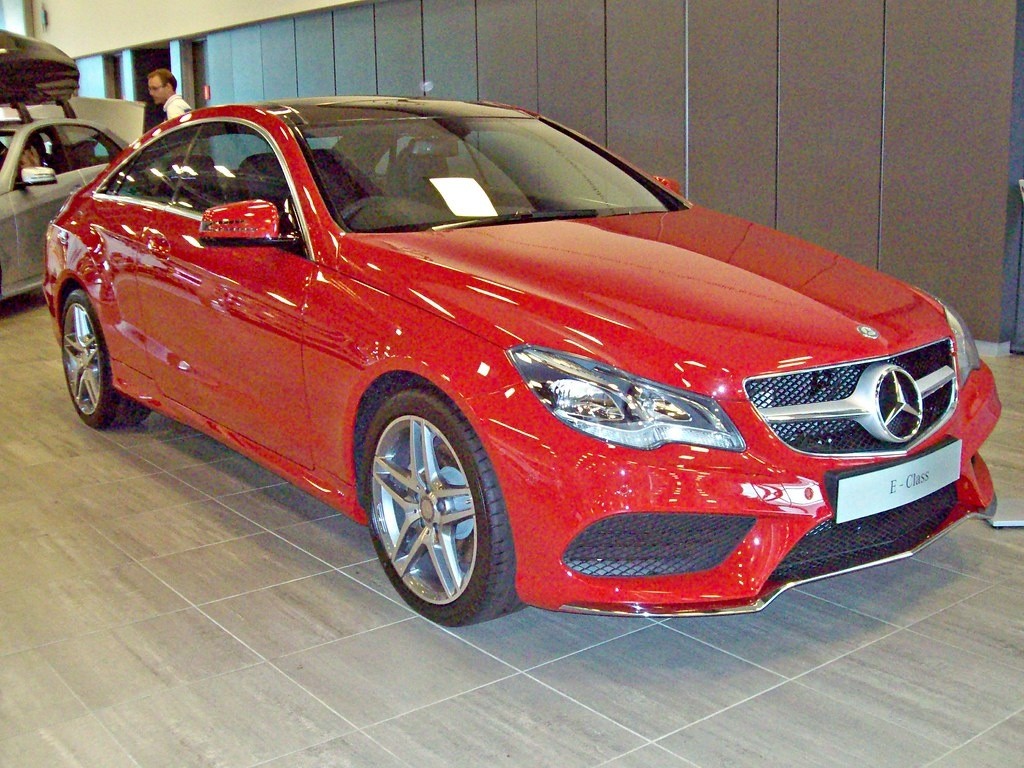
[158,152,223,206]
[387,148,449,207]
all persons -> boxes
[0,140,22,184]
[147,69,193,121]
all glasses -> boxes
[150,85,166,91]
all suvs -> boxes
[0,27,140,304]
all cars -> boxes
[41,96,1003,627]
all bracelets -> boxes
[1,148,6,155]
